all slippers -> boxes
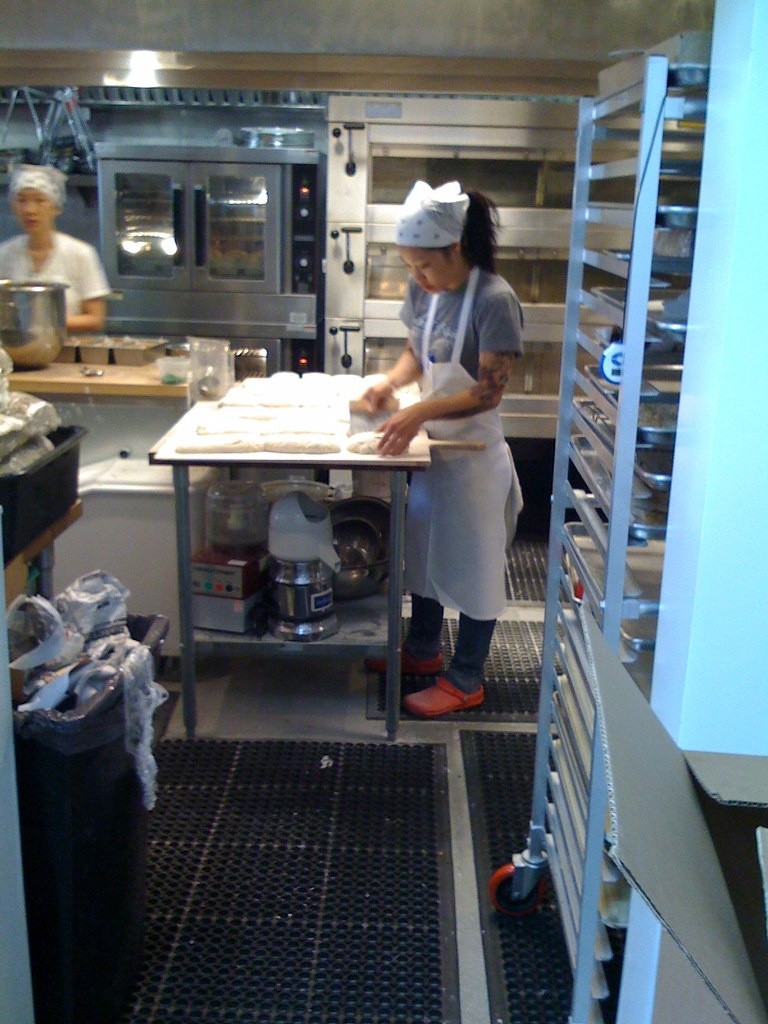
[403,673,484,719]
[364,639,443,674]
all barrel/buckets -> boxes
[0,280,71,368]
[206,481,268,555]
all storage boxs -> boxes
[0,424,85,569]
[578,604,768,1024]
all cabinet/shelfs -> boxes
[489,52,707,1024]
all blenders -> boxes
[269,490,339,641]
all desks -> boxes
[4,362,429,745]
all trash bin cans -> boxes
[11,613,171,1023]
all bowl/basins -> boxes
[166,344,188,356]
[328,563,393,599]
[330,494,392,564]
[155,356,190,384]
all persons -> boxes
[361,179,524,716]
[0,164,111,336]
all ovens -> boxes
[93,144,319,338]
[325,96,701,439]
